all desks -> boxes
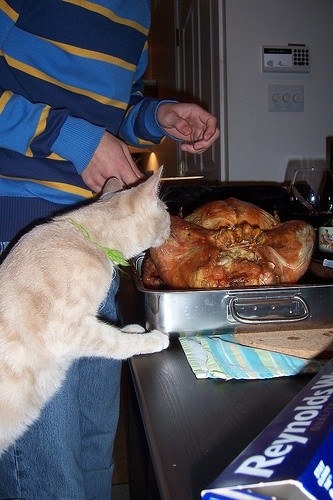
[115,180,333,500]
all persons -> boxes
[0,0,220,500]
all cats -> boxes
[0,165,171,455]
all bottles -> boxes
[313,135,333,264]
